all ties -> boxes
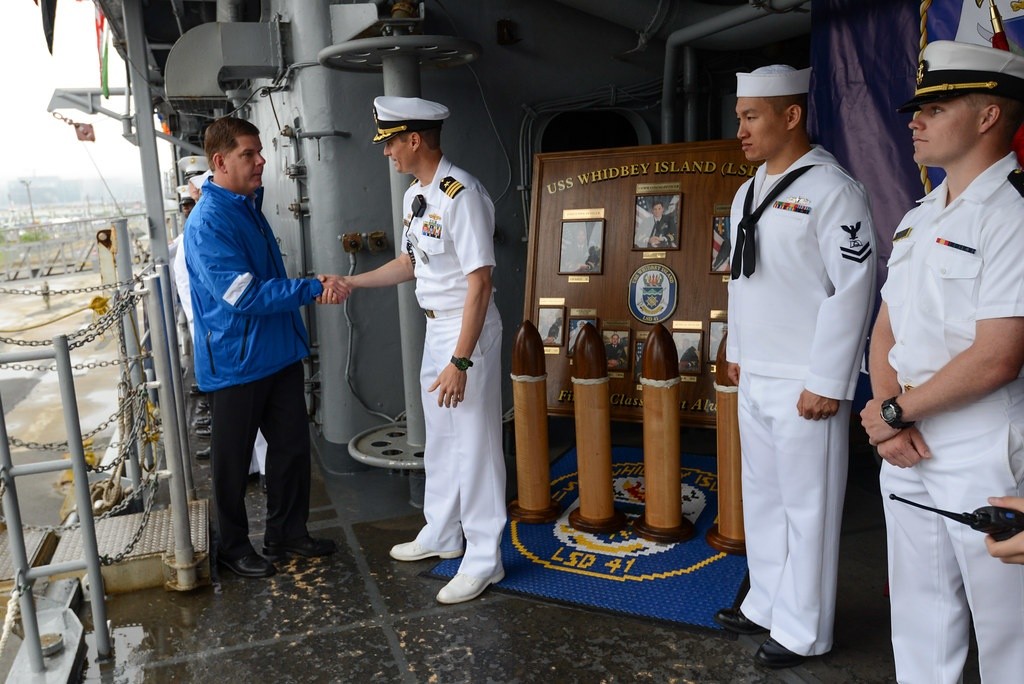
[730,166,818,279]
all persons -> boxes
[541,316,559,341]
[639,201,677,247]
[565,228,599,273]
[184,117,351,577]
[984,496,1024,566]
[175,157,268,494]
[716,65,876,669]
[316,97,507,604]
[860,39,1024,684]
[606,334,627,368]
[569,321,586,351]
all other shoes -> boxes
[194,441,211,459]
[189,396,213,437]
[389,539,462,561]
[436,569,504,603]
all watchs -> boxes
[880,396,916,429]
[451,356,474,371]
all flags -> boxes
[40,0,57,55]
[158,113,170,135]
[94,7,110,99]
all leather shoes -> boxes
[214,552,277,578]
[262,533,338,557]
[754,635,816,670]
[715,606,772,635]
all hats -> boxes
[370,95,450,145]
[175,185,195,205]
[189,170,213,192]
[896,40,1024,113]
[177,155,209,178]
[736,65,813,97]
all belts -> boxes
[895,374,1024,402]
